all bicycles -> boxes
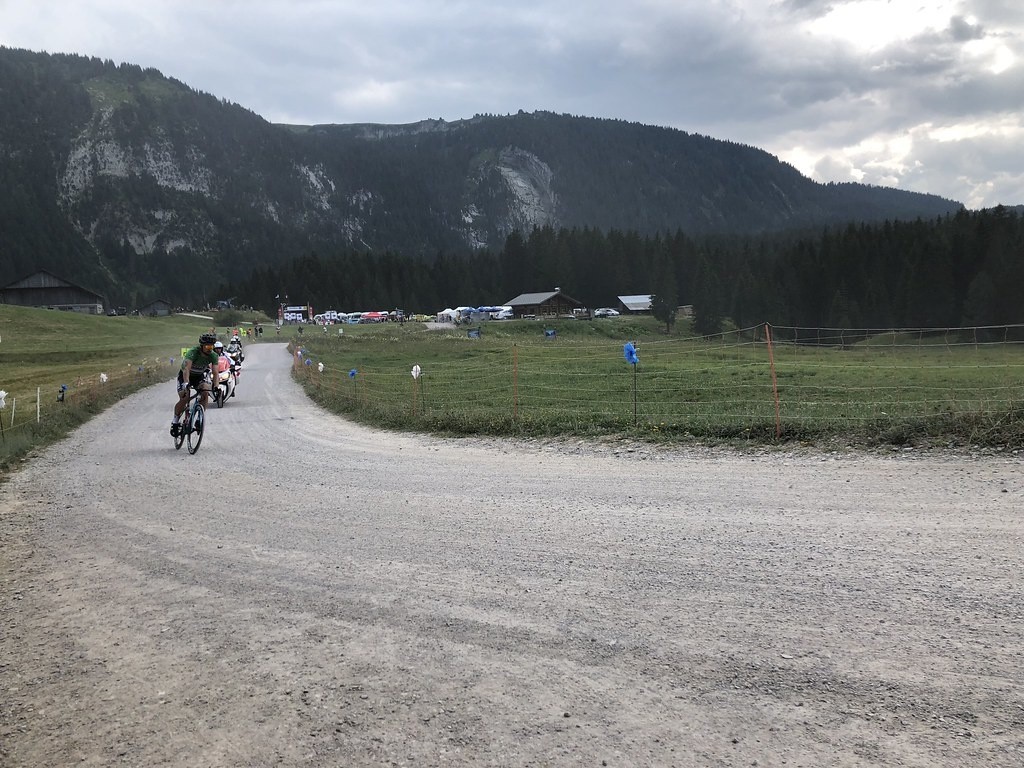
[171,384,222,455]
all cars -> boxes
[595,307,619,316]
[107,308,117,316]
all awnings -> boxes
[362,311,385,323]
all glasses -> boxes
[203,344,214,349]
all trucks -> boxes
[117,307,127,316]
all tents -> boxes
[435,304,513,324]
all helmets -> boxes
[213,341,224,353]
[230,339,236,344]
[199,333,216,345]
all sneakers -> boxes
[194,419,200,431]
[170,419,180,437]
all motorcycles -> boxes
[204,344,245,409]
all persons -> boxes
[223,322,264,364]
[276,326,280,334]
[170,332,220,437]
[298,326,304,334]
[211,341,234,376]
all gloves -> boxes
[213,387,222,397]
[181,382,195,393]
